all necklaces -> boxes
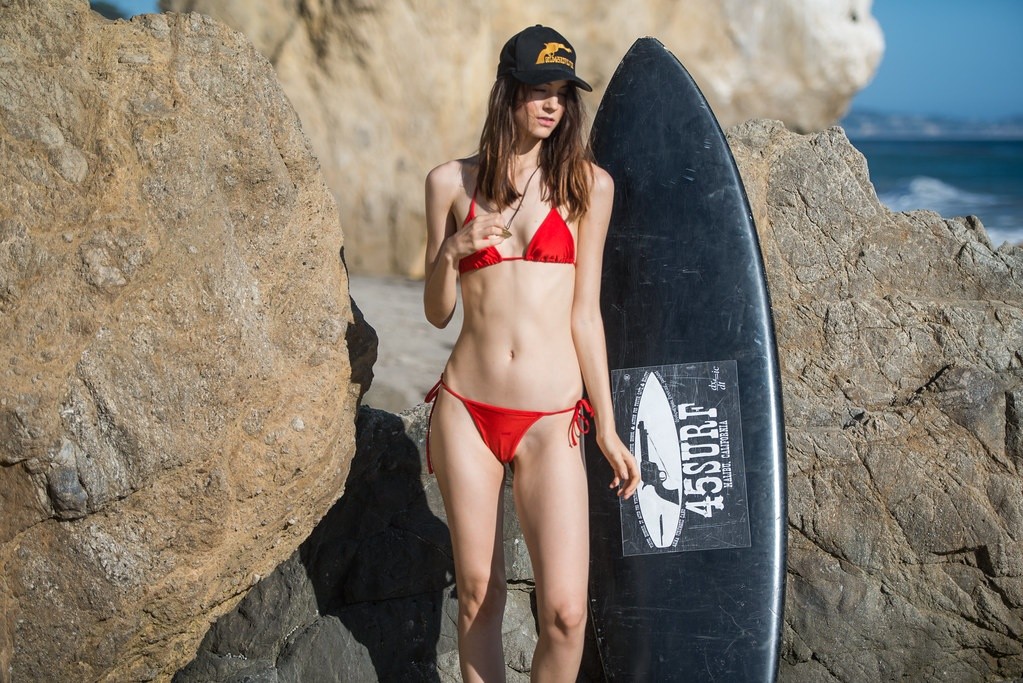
[495,159,544,230]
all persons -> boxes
[424,22,644,683]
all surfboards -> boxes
[574,36,789,683]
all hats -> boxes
[497,24,593,92]
